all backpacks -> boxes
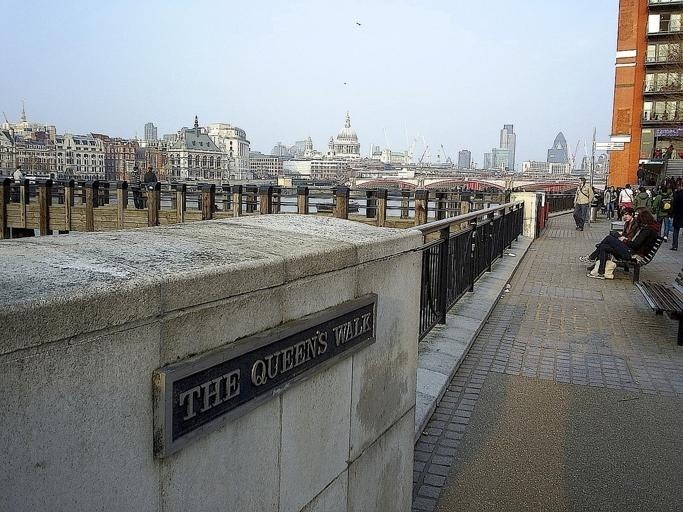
[659,193,672,212]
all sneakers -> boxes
[579,254,595,263]
[663,236,678,251]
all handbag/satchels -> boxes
[589,259,617,280]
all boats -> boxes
[314,199,359,213]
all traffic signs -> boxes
[594,146,624,150]
[594,142,624,147]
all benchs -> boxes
[610,235,683,347]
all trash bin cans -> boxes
[589,203,598,223]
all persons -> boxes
[636,166,645,185]
[129,166,143,209]
[667,179,683,251]
[578,206,660,280]
[587,206,640,270]
[573,177,594,232]
[651,185,670,242]
[12,166,24,183]
[592,174,683,222]
[663,144,673,159]
[143,167,157,182]
